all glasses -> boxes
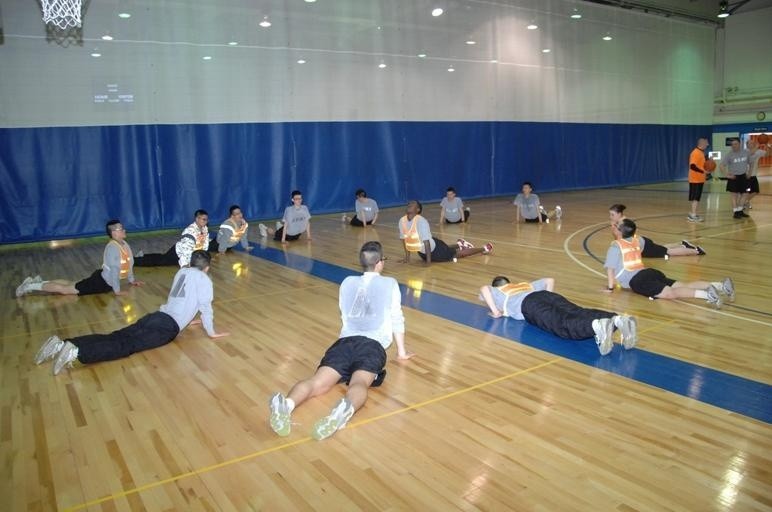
[197,216,209,221]
[375,255,388,263]
[291,197,302,200]
[230,211,242,216]
[112,226,123,232]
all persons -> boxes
[607,204,707,258]
[16,221,146,298]
[132,209,209,271]
[510,183,563,225]
[435,187,471,226]
[269,241,418,442]
[478,276,638,356]
[208,205,254,256]
[743,140,769,209]
[258,190,312,244]
[600,218,736,309]
[341,189,379,228]
[719,138,763,219]
[685,137,710,223]
[396,199,495,266]
[34,250,231,375]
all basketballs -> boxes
[758,134,769,144]
[704,160,716,171]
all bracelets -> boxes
[608,287,614,290]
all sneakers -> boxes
[594,318,613,355]
[538,205,547,214]
[482,242,493,253]
[457,238,473,250]
[32,275,43,283]
[312,396,353,440]
[33,334,63,365]
[742,201,753,209]
[685,212,704,222]
[259,224,267,236]
[269,391,291,437]
[276,222,282,230]
[556,205,562,219]
[52,340,78,376]
[16,276,33,296]
[464,207,469,211]
[681,240,695,249]
[618,317,638,350]
[721,277,735,302]
[733,212,741,218]
[741,211,749,217]
[705,284,721,309]
[695,245,706,255]
